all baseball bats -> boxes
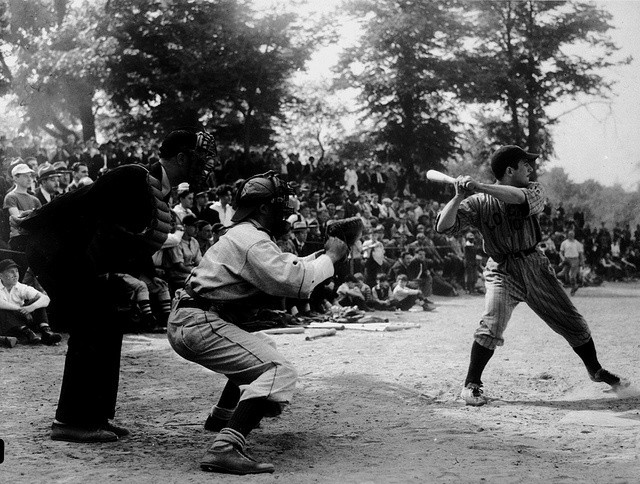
[426,169,475,191]
[304,325,345,330]
[306,329,336,340]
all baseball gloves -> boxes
[326,217,363,261]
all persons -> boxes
[167,170,350,475]
[232,293,281,328]
[101,238,166,330]
[620,223,640,280]
[34,131,92,209]
[281,171,350,328]
[434,146,620,407]
[9,136,34,283]
[92,132,161,182]
[217,148,350,171]
[0,136,8,248]
[434,181,487,297]
[0,259,62,345]
[351,154,434,320]
[167,181,220,319]
[221,177,245,235]
[9,129,221,443]
[537,196,620,297]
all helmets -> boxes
[231,170,295,238]
[159,128,221,185]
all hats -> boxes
[490,145,541,164]
[53,161,74,173]
[12,164,36,175]
[182,215,206,226]
[212,222,227,232]
[37,162,63,180]
[0,259,19,270]
[8,157,33,177]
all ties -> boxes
[63,189,65,194]
[50,194,54,199]
[201,205,205,212]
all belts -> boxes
[175,299,213,310]
[489,246,538,260]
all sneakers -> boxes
[49,420,118,442]
[424,297,434,304]
[145,318,168,333]
[460,383,487,406]
[200,443,273,474]
[21,329,42,344]
[41,333,62,345]
[101,421,129,437]
[203,413,262,429]
[589,368,631,389]
[424,304,434,311]
